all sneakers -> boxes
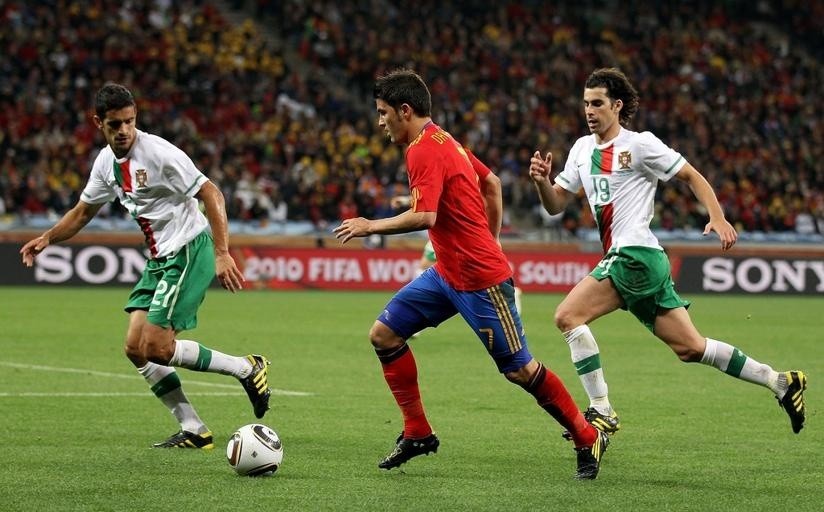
[378,431,440,470]
[562,407,622,441]
[573,427,610,483]
[239,354,271,419]
[152,431,215,450]
[778,370,807,434]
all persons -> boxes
[329,68,612,481]
[526,66,810,441]
[19,82,274,449]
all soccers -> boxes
[225,423,282,475]
